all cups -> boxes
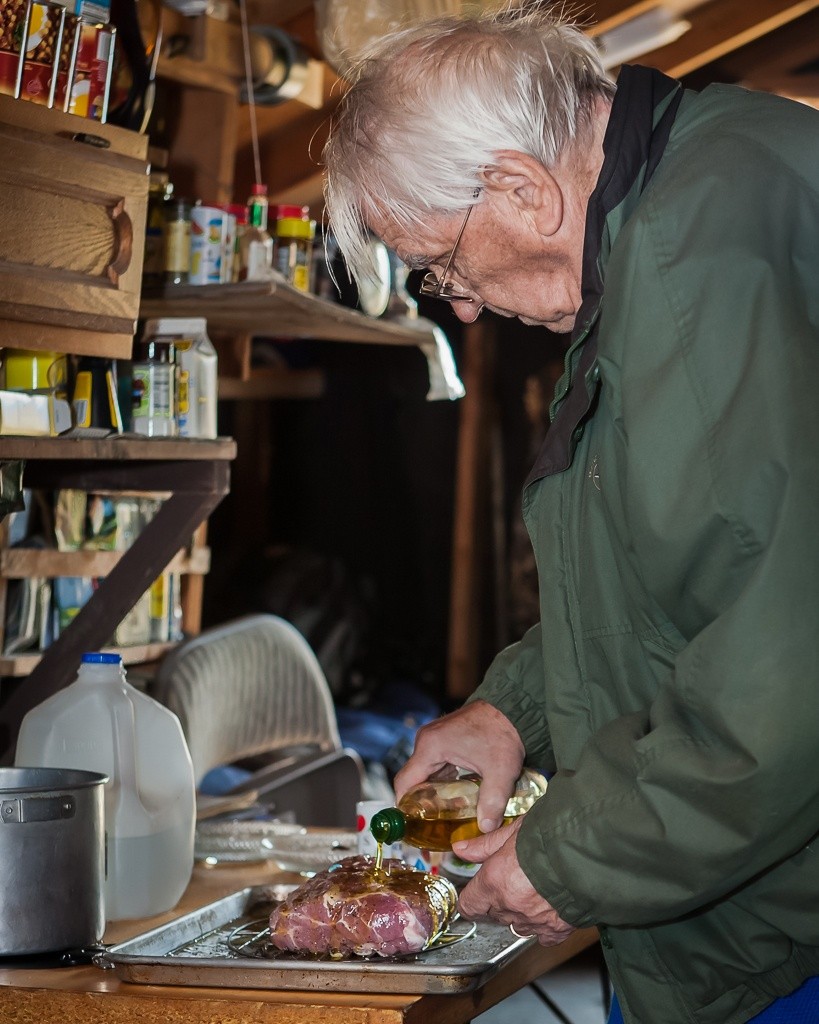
[159,193,250,286]
[356,800,401,862]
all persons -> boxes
[333,10,819,1024]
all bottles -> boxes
[239,183,274,286]
[275,204,318,293]
[71,355,133,433]
[370,764,547,852]
[16,653,197,922]
[132,342,179,440]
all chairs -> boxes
[157,614,363,828]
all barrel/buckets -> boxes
[0,765,114,969]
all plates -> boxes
[194,822,308,864]
[260,835,362,878]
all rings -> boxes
[509,924,534,939]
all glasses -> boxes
[419,171,486,303]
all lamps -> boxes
[599,6,692,72]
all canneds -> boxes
[125,338,178,439]
[188,200,317,293]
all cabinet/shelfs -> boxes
[0,0,600,1024]
